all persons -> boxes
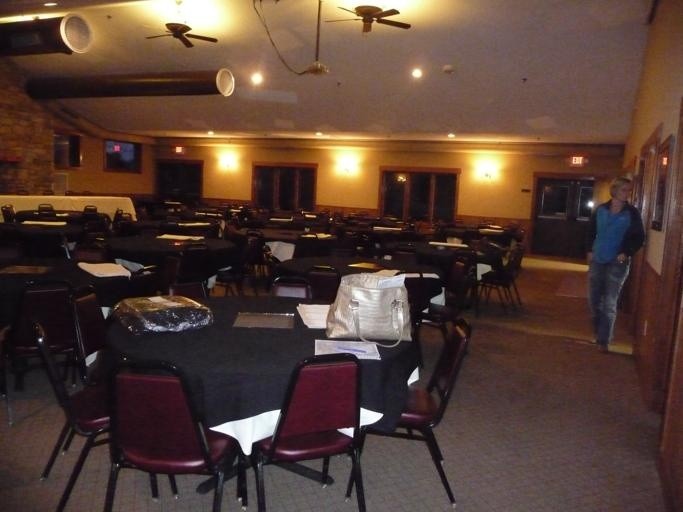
[579,177,646,356]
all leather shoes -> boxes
[593,335,610,354]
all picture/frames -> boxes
[648,135,675,233]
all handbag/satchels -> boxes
[111,293,214,336]
[326,269,413,349]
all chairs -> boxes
[0,201,527,512]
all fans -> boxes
[324,0,411,33]
[144,0,218,48]
